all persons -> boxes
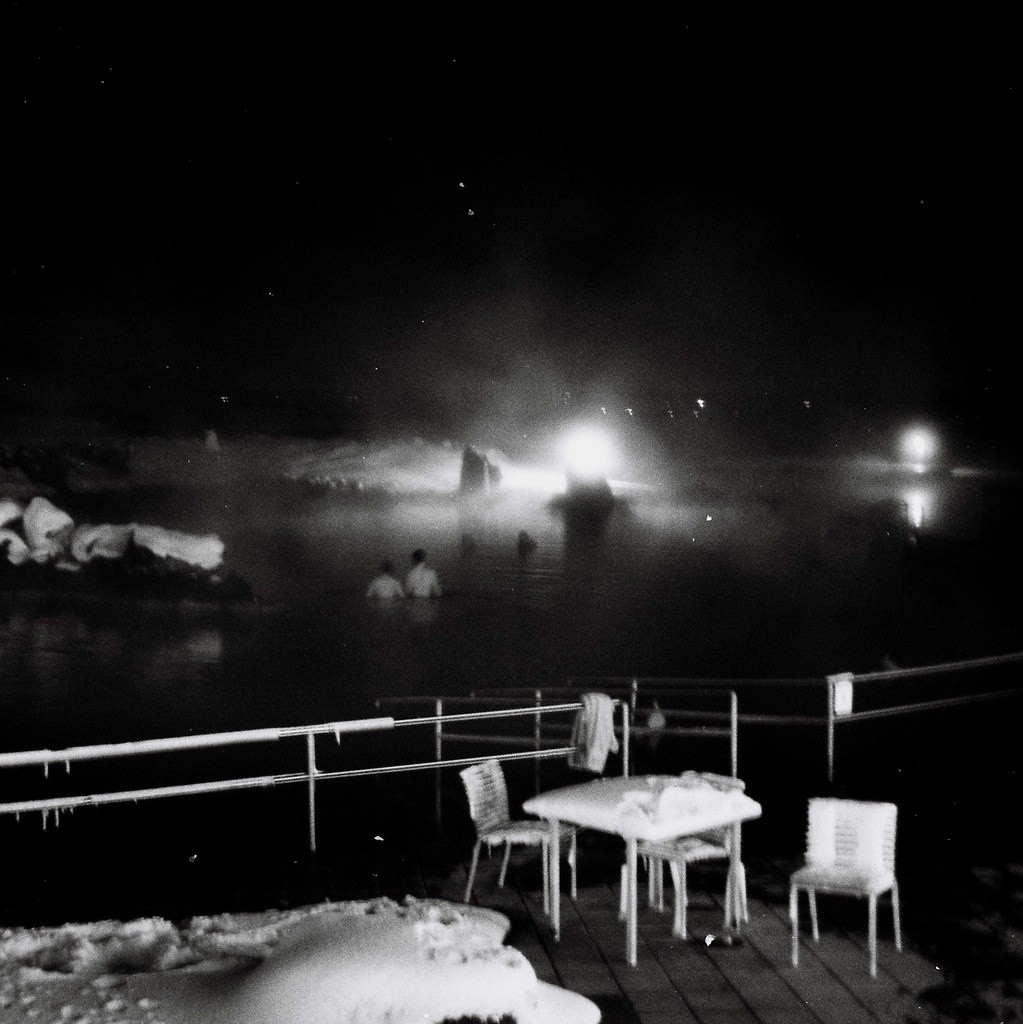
[406,548,444,600]
[364,560,408,601]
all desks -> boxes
[523,776,761,966]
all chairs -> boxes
[790,798,901,978]
[620,771,745,938]
[459,759,576,914]
